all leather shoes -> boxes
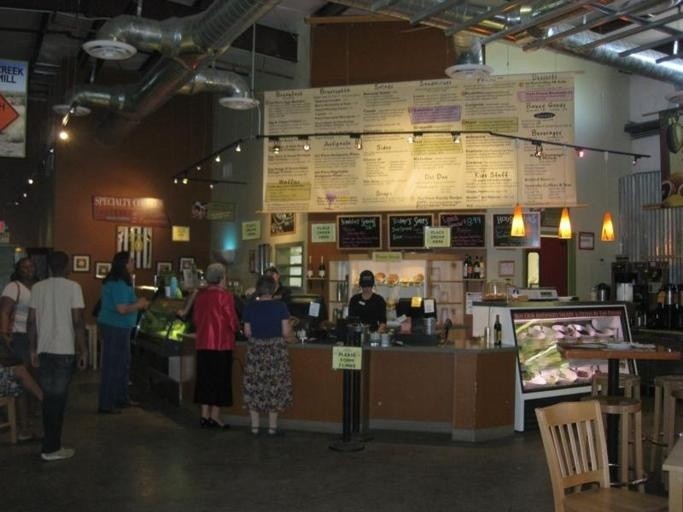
[93,295,102,319]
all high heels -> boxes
[207,416,232,430]
[199,417,207,427]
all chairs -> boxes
[0,395,19,443]
[534,399,667,512]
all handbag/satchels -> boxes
[0,280,21,336]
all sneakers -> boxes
[98,396,139,414]
[18,428,75,461]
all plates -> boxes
[562,337,656,351]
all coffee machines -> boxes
[611,254,671,330]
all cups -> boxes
[368,333,380,347]
[164,286,170,298]
[380,333,391,348]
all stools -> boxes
[647,376,683,489]
[580,396,645,490]
[580,372,640,466]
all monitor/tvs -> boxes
[395,298,437,332]
[288,294,329,328]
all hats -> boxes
[358,269,374,287]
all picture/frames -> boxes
[179,257,195,272]
[72,255,91,273]
[156,260,173,275]
[579,231,594,250]
[94,261,112,280]
[499,260,514,277]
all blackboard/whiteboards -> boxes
[337,211,541,250]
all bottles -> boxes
[430,267,450,326]
[331,282,348,326]
[306,255,314,279]
[170,277,179,298]
[503,278,512,305]
[493,314,502,346]
[318,255,327,280]
[463,255,485,280]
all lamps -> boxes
[558,143,573,239]
[600,150,616,241]
[511,137,526,237]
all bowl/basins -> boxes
[518,322,614,388]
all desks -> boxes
[662,436,683,512]
[557,341,683,486]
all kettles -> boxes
[596,282,611,302]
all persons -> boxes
[348,271,386,333]
[0,257,38,367]
[192,262,240,430]
[264,267,292,307]
[26,251,88,460]
[241,274,293,437]
[0,365,46,443]
[97,251,146,414]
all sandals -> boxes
[249,424,261,436]
[266,425,285,439]
